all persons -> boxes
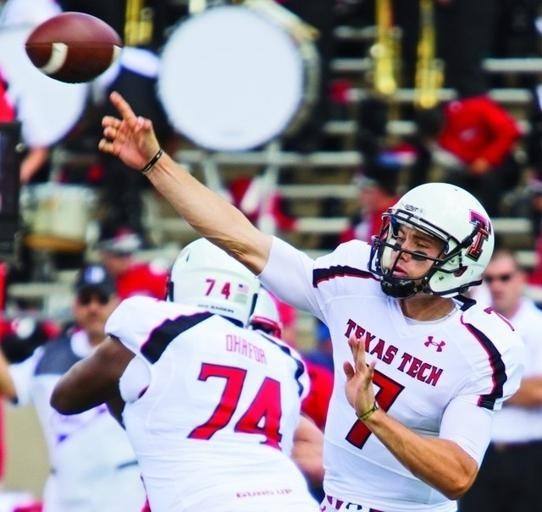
[1,1,541,511]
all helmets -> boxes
[367,182,495,298]
[250,285,282,340]
[165,235,260,327]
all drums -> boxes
[18,181,103,254]
[158,0,324,152]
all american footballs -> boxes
[25,13,123,83]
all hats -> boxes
[77,264,113,291]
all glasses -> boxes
[80,293,108,305]
